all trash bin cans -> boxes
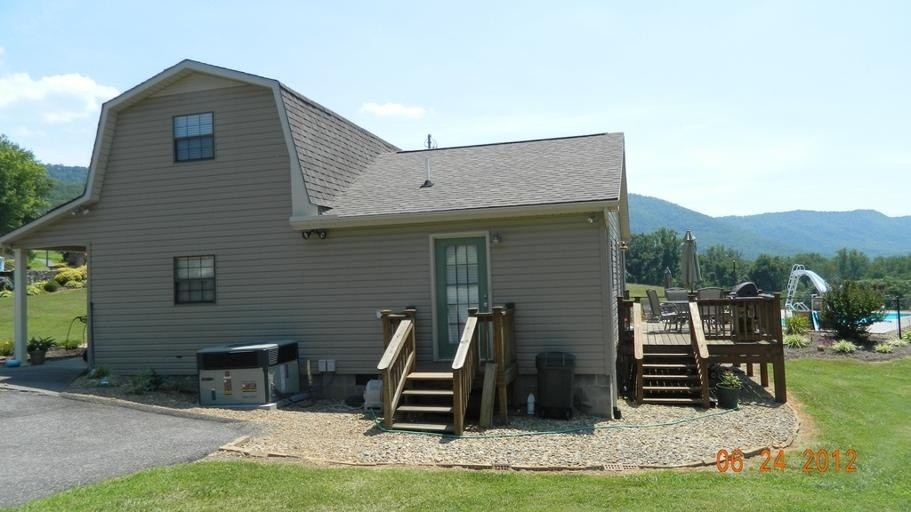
[537,353,575,420]
[728,281,762,342]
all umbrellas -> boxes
[682,228,706,293]
[664,267,673,296]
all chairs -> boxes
[645,286,725,335]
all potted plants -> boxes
[714,371,742,410]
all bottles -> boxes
[526,392,535,415]
